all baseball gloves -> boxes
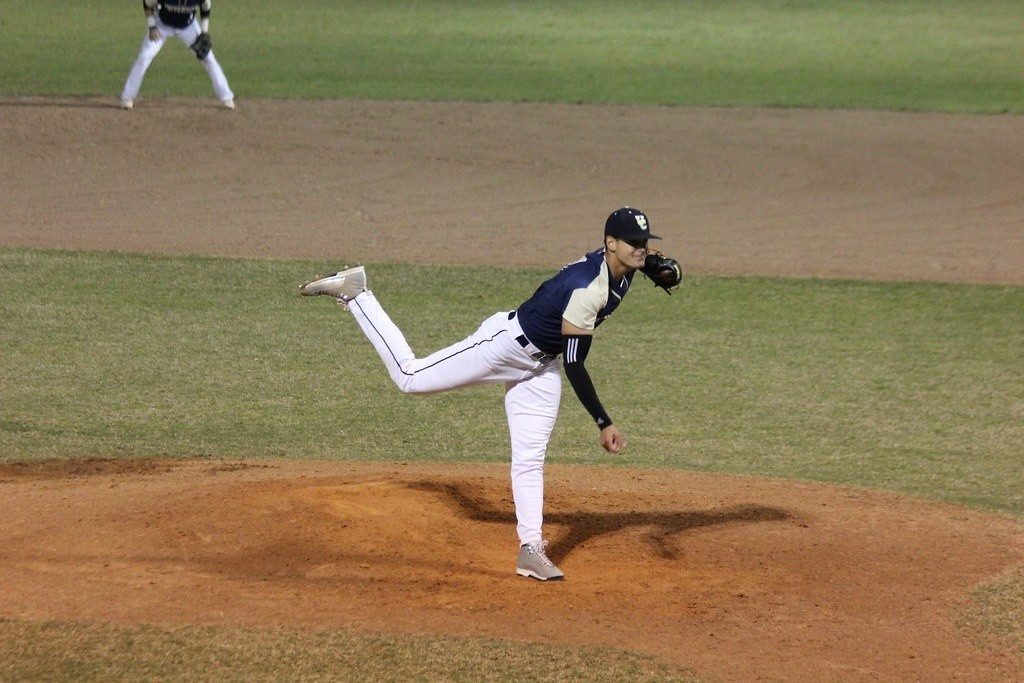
[191,32,212,62]
[644,254,682,296]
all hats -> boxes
[604,206,662,241]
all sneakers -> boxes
[223,99,237,110]
[121,99,134,109]
[298,264,366,301]
[516,544,564,580]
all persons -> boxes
[301,207,682,583]
[123,2,235,112]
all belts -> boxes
[508,311,557,364]
